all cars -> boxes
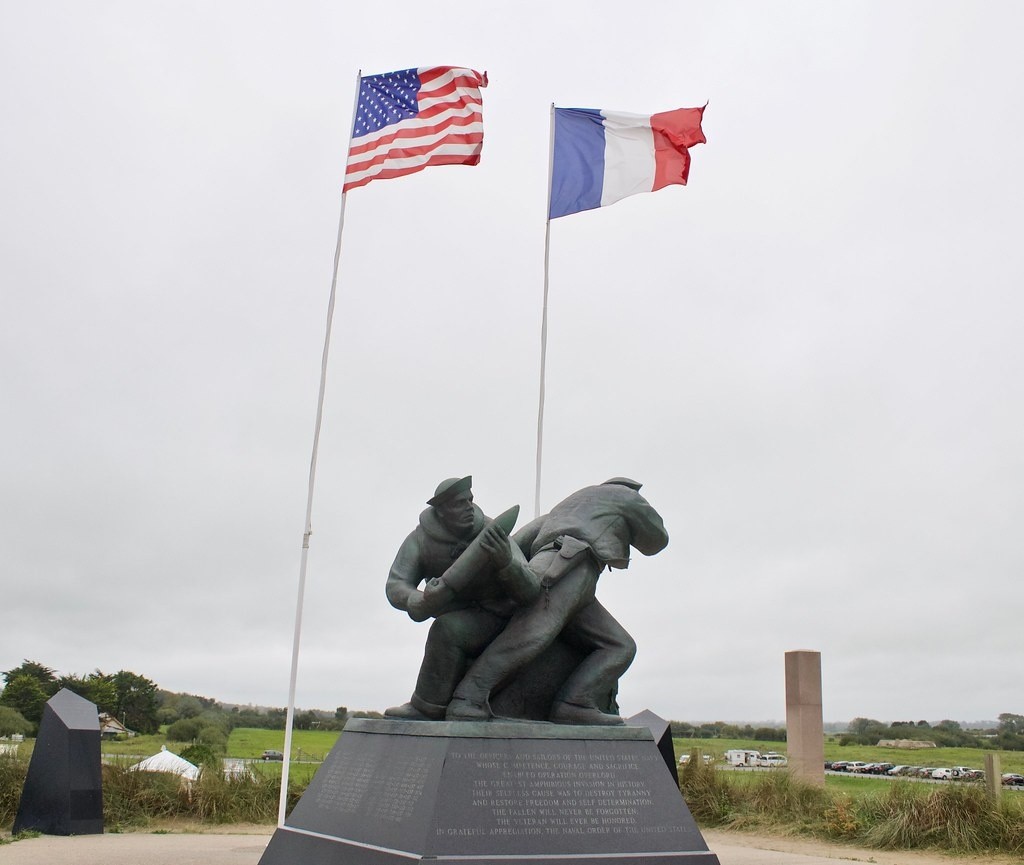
[679,755,689,767]
[999,773,1024,785]
[703,755,714,766]
[824,761,984,781]
[760,754,787,768]
[262,749,285,761]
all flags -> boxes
[546,100,708,219]
[341,65,489,190]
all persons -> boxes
[387,474,668,727]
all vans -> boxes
[724,749,762,767]
